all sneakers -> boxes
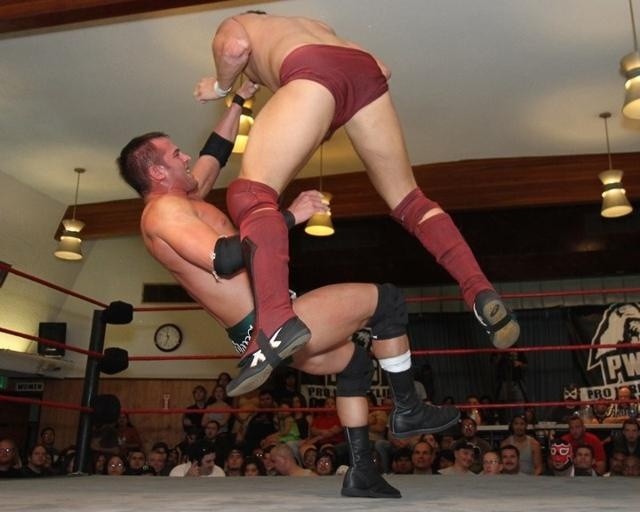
[225,315,311,399]
[473,289,520,350]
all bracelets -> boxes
[212,79,233,99]
[230,94,246,109]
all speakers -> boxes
[38,322,67,358]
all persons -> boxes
[208,372,237,423]
[275,375,306,405]
[420,353,536,425]
[194,10,521,397]
[2,387,638,477]
[114,131,462,499]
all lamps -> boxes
[596,112,634,219]
[222,73,256,155]
[54,166,87,262]
[305,137,336,239]
[621,0,640,121]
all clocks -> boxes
[153,323,183,352]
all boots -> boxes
[381,360,461,438]
[341,424,401,498]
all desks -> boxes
[475,421,625,474]
